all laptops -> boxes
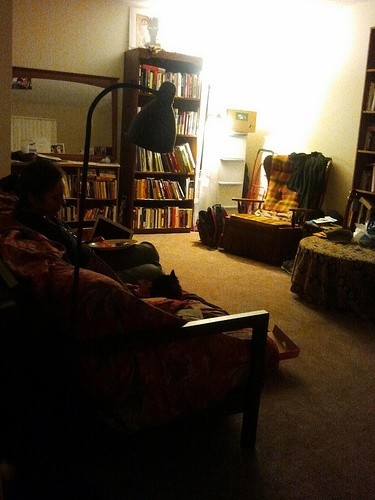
[89,214,134,242]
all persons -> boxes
[11,159,163,284]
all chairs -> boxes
[0,221,269,500]
[223,156,332,268]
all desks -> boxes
[290,236,375,322]
[88,239,137,251]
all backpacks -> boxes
[199,206,224,250]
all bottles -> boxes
[92,235,112,249]
[29,143,37,152]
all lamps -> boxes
[68,83,175,329]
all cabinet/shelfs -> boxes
[343,29,375,195]
[11,48,204,234]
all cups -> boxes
[22,140,29,153]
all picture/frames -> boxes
[51,143,66,153]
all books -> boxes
[58,168,117,221]
[132,64,196,229]
[348,81,375,224]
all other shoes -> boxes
[281,259,295,274]
